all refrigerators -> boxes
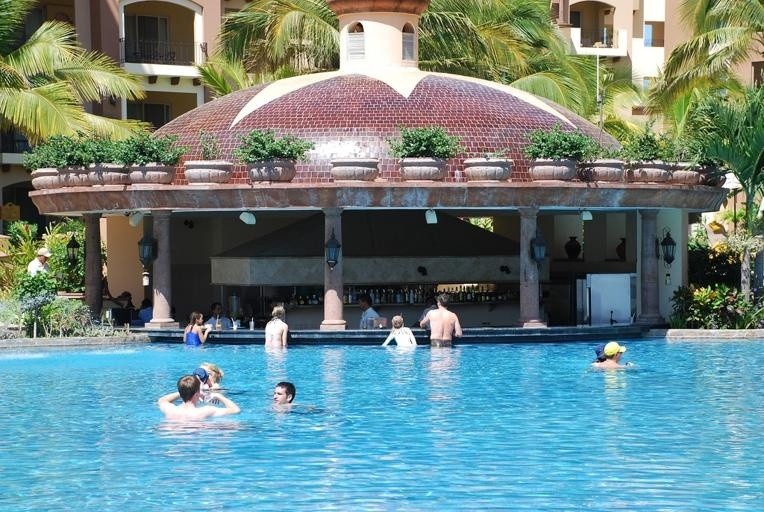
[584,271,637,326]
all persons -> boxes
[25,247,53,275]
[156,375,242,421]
[589,342,639,370]
[272,381,296,407]
[592,346,607,364]
[197,363,224,389]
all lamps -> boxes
[324,227,341,271]
[418,266,427,276]
[138,227,158,286]
[501,266,512,274]
[580,211,593,221]
[425,209,437,224]
[656,231,676,285]
[128,211,145,228]
[66,234,86,266]
[531,229,548,267]
[239,212,256,224]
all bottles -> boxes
[294,287,521,304]
[367,316,374,330]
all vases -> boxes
[616,238,625,261]
[565,236,581,260]
[329,158,380,180]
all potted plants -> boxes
[384,123,468,180]
[231,128,316,181]
[519,118,732,187]
[21,128,191,189]
[184,128,234,183]
[463,146,514,180]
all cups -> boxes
[215,319,221,330]
[232,321,237,330]
[249,321,254,330]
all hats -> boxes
[604,341,626,356]
[595,344,605,358]
